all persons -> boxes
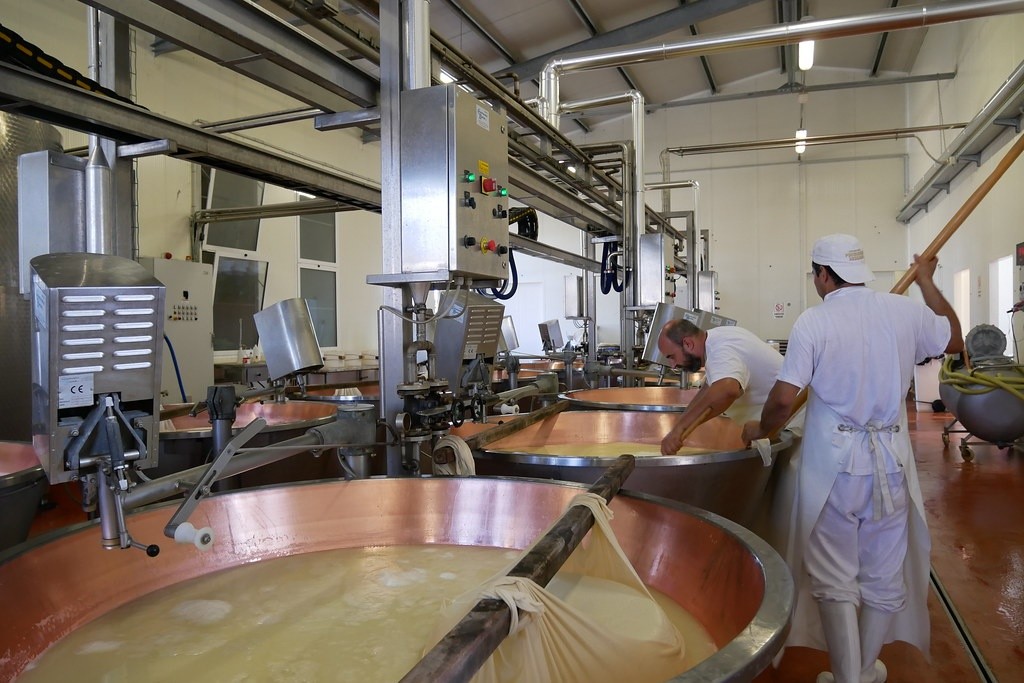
[658,318,807,456]
[743,233,963,683]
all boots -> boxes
[815,599,860,683]
[859,602,888,683]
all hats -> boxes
[812,234,876,285]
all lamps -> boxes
[794,127,807,154]
[798,13,814,70]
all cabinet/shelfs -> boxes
[305,365,378,384]
[213,361,269,384]
